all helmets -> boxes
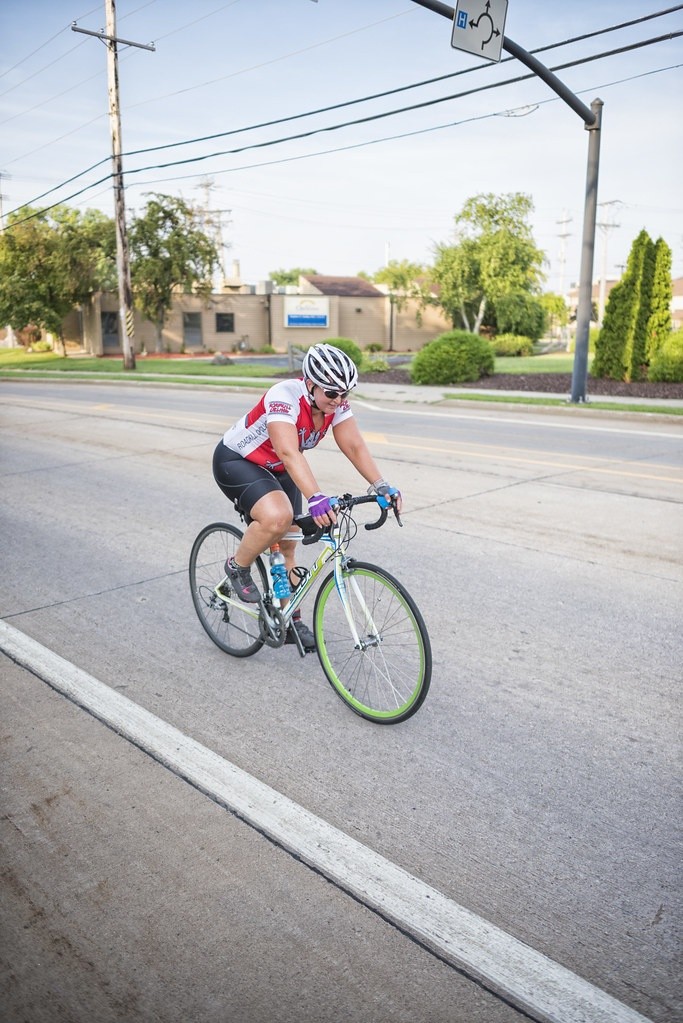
[302,344,359,391]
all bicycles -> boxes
[189,493,432,725]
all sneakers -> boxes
[224,556,262,603]
[284,620,326,649]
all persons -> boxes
[213,344,402,649]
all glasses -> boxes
[318,385,349,401]
